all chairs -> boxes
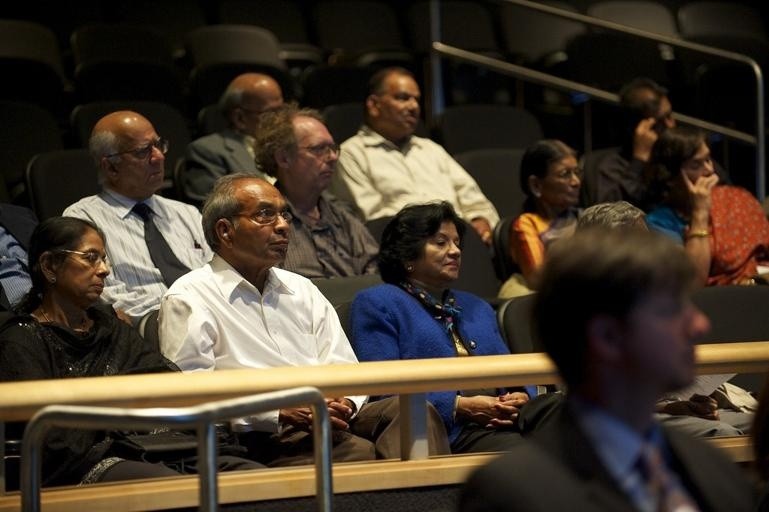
[2,3,766,349]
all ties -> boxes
[638,438,701,512]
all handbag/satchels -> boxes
[111,428,202,463]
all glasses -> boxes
[538,168,584,181]
[285,141,343,158]
[50,246,108,267]
[106,134,173,160]
[227,206,294,226]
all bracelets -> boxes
[684,228,710,240]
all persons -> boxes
[150,169,453,466]
[582,74,733,218]
[329,64,502,241]
[450,223,768,512]
[58,109,219,352]
[170,68,290,211]
[0,214,271,487]
[248,98,382,281]
[642,125,769,291]
[0,208,42,326]
[570,196,757,442]
[508,137,583,294]
[348,197,566,456]
